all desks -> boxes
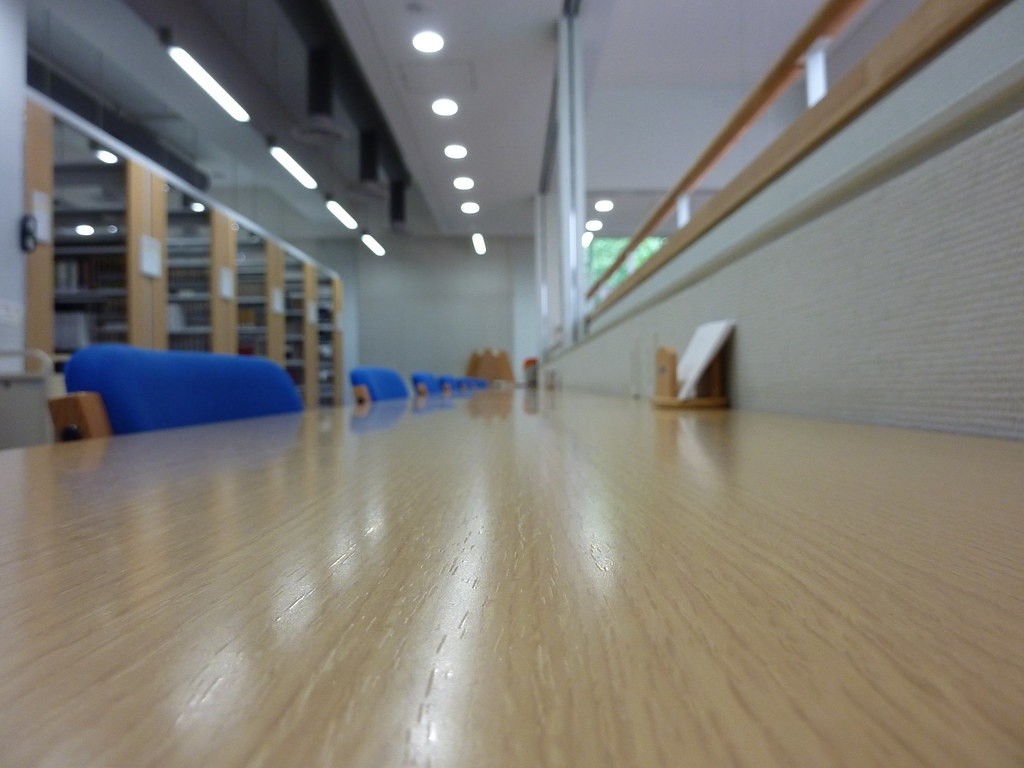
[0,387,1024,768]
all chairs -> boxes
[49,344,489,444]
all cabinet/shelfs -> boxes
[51,247,335,412]
